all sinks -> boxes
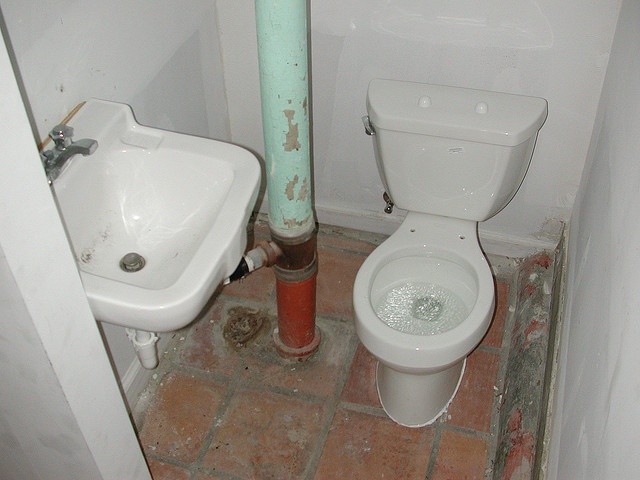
[51,98,263,333]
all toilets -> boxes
[353,77,548,428]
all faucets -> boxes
[40,124,98,187]
